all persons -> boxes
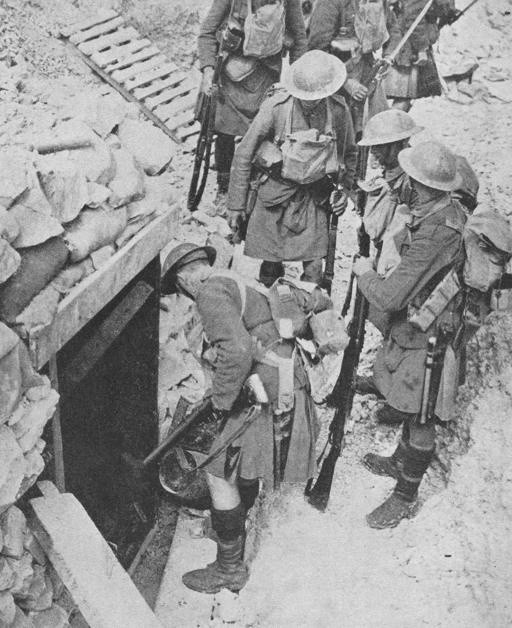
[355,110,460,428]
[353,142,469,530]
[161,243,320,595]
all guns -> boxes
[187,33,230,210]
[347,146,366,337]
[309,235,370,511]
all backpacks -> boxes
[196,269,336,340]
[415,196,511,294]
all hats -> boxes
[357,109,425,147]
[398,140,464,193]
[283,49,348,101]
[159,242,218,297]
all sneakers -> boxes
[365,486,423,530]
[181,559,250,595]
[362,452,409,483]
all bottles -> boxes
[330,26,353,63]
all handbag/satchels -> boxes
[242,2,287,60]
[278,127,341,185]
[356,176,398,246]
[354,1,391,56]
